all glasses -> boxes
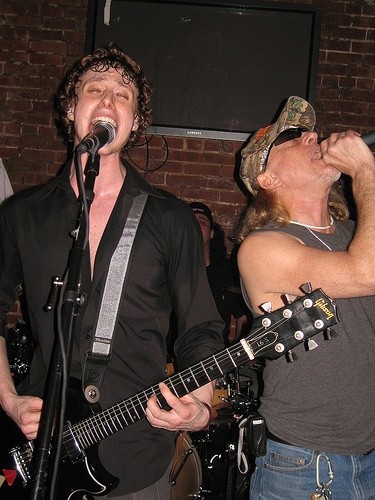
[274,127,308,146]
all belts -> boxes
[266,430,291,444]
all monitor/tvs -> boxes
[85,0,321,141]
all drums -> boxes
[164,361,241,426]
[81,428,204,500]
[236,376,253,416]
[4,322,35,368]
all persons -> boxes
[0,47,254,500]
[235,96,375,500]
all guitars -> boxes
[0,281,342,500]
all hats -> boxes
[242,97,316,197]
[189,202,214,227]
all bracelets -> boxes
[201,403,212,430]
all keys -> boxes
[311,493,326,500]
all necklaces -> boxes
[280,213,335,230]
[306,226,334,252]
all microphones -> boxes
[75,121,116,154]
[361,132,375,145]
